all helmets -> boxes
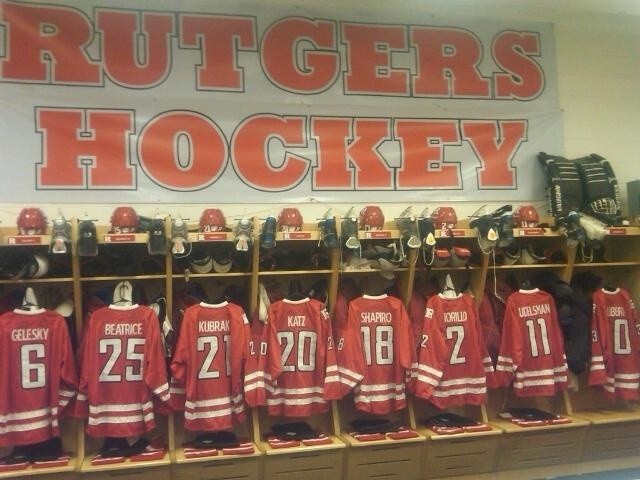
[200,208,225,232]
[513,205,540,228]
[520,245,547,264]
[580,240,606,263]
[279,207,302,232]
[18,207,47,234]
[359,205,384,229]
[110,206,138,233]
[20,253,50,279]
[189,250,235,274]
[432,206,457,229]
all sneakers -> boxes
[471,215,513,253]
[50,220,70,254]
[557,212,585,247]
[317,217,360,250]
[171,218,188,244]
[147,220,168,255]
[234,218,253,251]
[261,218,277,250]
[397,216,437,250]
[77,222,99,257]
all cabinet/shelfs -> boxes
[1,214,639,479]
